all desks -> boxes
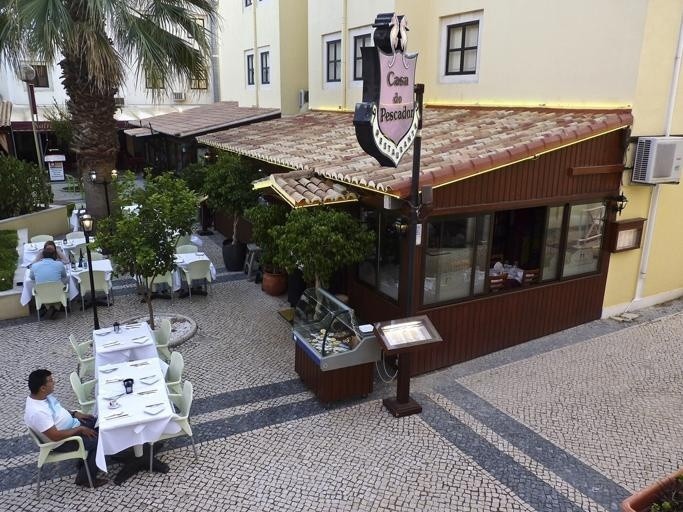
[69,208,85,230]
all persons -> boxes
[28,246,69,313]
[33,241,69,264]
[22,369,109,489]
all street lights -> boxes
[88,165,119,235]
[14,64,46,179]
[79,211,96,329]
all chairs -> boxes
[19,231,211,322]
[449,252,540,297]
[25,319,199,498]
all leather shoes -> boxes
[76,477,107,487]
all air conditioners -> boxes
[171,91,185,101]
[631,136,682,184]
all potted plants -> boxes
[203,154,286,295]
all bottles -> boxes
[69,247,88,270]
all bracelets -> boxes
[71,411,77,418]
